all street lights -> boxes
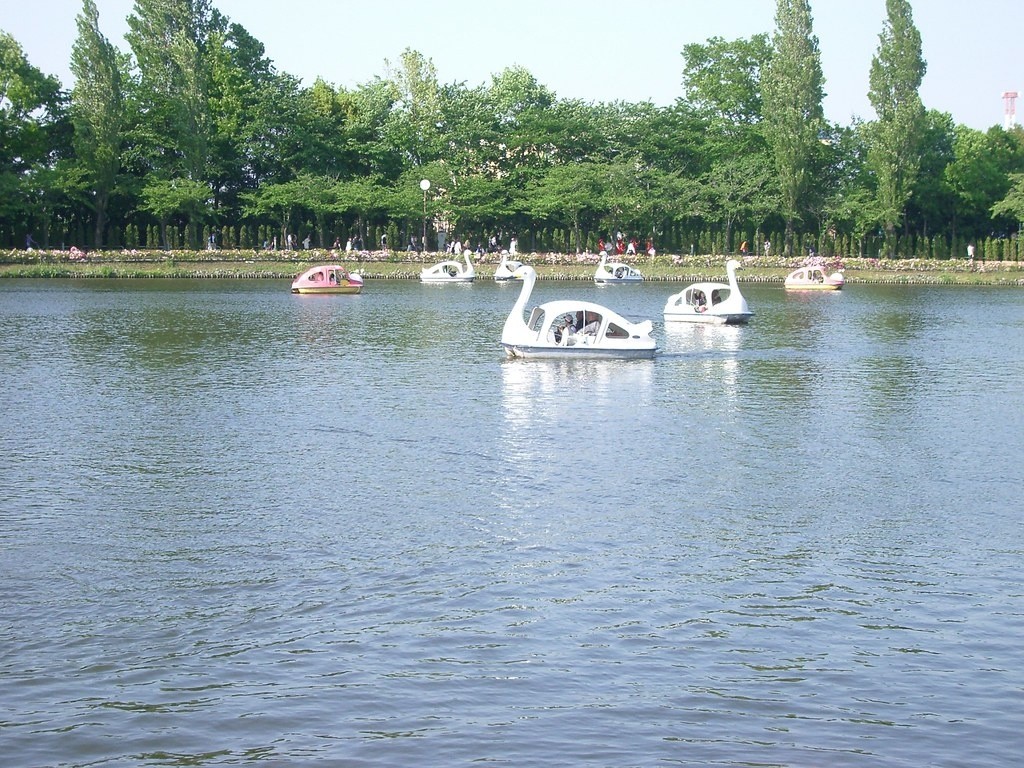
[419,179,431,251]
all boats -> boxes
[662,258,755,324]
[420,249,476,283]
[291,264,365,295]
[594,250,644,284]
[501,264,658,352]
[783,264,844,290]
[491,249,528,283]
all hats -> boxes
[564,315,573,322]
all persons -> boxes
[26,232,35,249]
[208,231,216,250]
[264,231,846,341]
[967,244,974,260]
